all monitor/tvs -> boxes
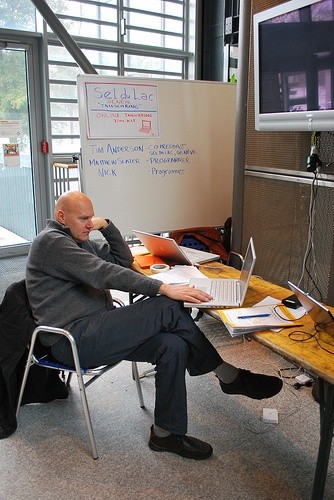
[253,0,334,132]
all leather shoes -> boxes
[215,367,284,400]
[148,424,213,460]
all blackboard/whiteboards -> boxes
[77,74,237,240]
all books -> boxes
[217,303,304,338]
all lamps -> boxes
[307,131,322,175]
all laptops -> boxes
[132,230,220,266]
[184,236,257,307]
[288,281,334,339]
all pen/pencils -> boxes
[238,314,271,319]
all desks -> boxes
[127,241,334,500]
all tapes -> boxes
[150,264,170,273]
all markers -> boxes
[170,265,199,269]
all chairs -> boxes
[6,285,144,458]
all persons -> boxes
[25,192,283,460]
